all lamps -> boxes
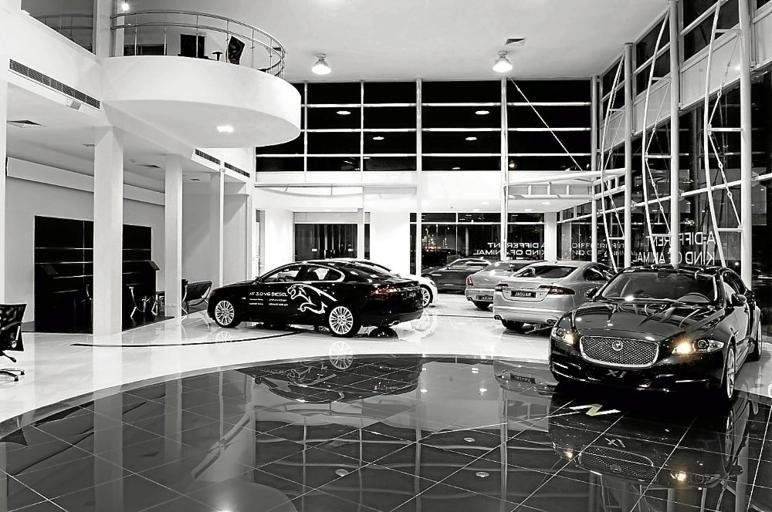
[491,51,512,73]
[311,53,331,74]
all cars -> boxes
[207,257,439,338]
[421,257,492,291]
[492,260,618,331]
[547,263,763,411]
[465,260,549,309]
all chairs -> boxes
[1,303,27,381]
[84,279,212,329]
